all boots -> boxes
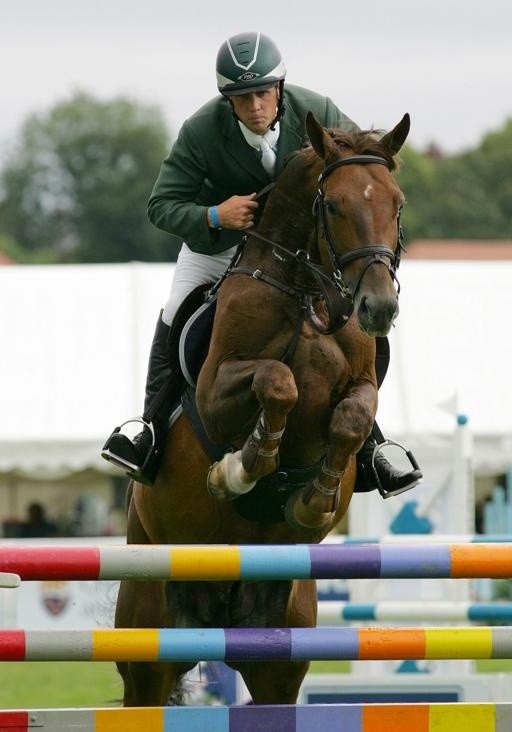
[109,307,172,486]
[351,435,422,493]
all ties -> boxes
[259,139,277,179]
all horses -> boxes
[114,110,411,707]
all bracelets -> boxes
[209,205,222,233]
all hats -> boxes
[215,32,286,96]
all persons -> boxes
[100,33,423,495]
[8,503,63,539]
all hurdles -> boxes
[0,542,511,732]
